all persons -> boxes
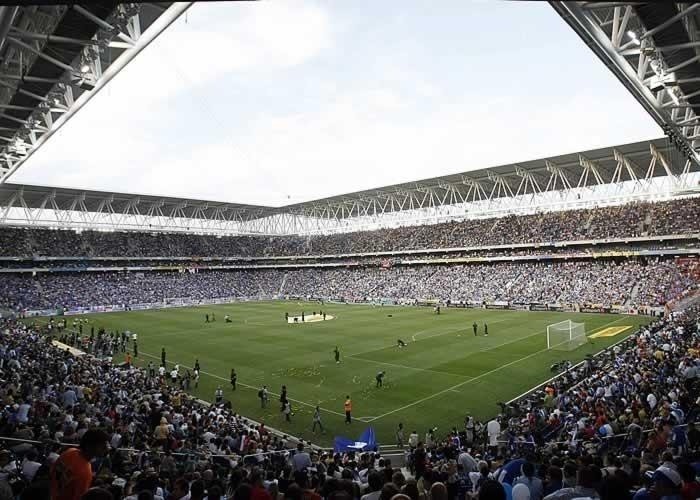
[0,197,700,499]
